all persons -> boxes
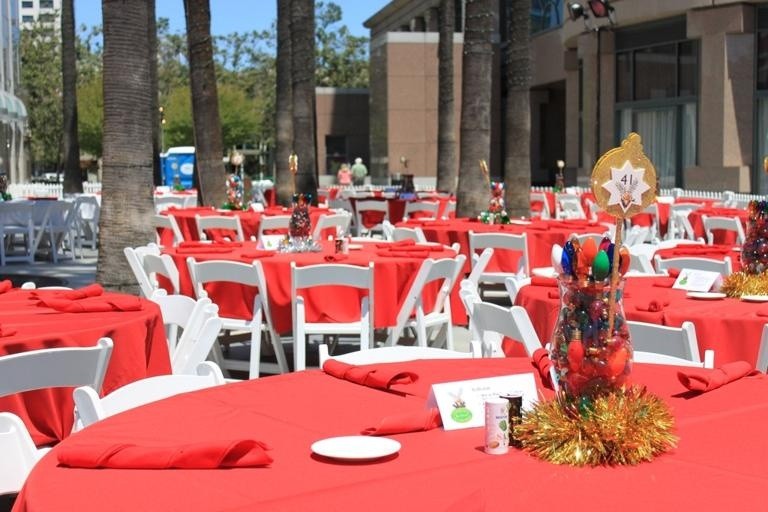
[337,163,353,185]
[350,157,368,186]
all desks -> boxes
[15,353,766,511]
[0,280,163,496]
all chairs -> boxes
[124,184,767,382]
[72,360,225,426]
[0,192,100,266]
[1,338,114,426]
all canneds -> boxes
[342,238,349,254]
[499,394,522,446]
[485,399,509,454]
[335,240,342,254]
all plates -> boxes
[349,244,362,249]
[310,435,401,460]
[687,292,768,303]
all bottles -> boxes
[335,238,349,254]
[484,394,523,455]
[549,276,633,409]
[740,209,768,274]
